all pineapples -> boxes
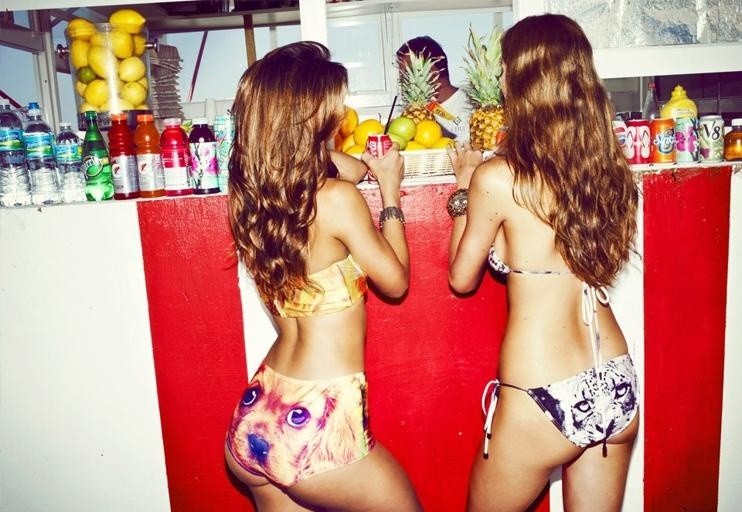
[458,22,506,150]
[393,42,444,124]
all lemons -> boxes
[67,10,150,110]
[339,108,366,156]
[387,115,454,149]
[354,118,382,147]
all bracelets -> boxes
[378,206,408,231]
[447,186,470,218]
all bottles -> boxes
[0,99,32,207]
[641,83,660,120]
[189,117,217,195]
[82,110,114,201]
[214,116,235,194]
[55,123,85,204]
[26,101,61,206]
[725,118,742,161]
[661,84,699,120]
[108,114,138,199]
[134,115,164,198]
[160,119,189,196]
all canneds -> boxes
[650,119,676,166]
[627,119,652,164]
[676,117,701,163]
[366,135,393,184]
[612,121,627,158]
[699,115,725,163]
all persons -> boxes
[222,40,425,512]
[394,35,475,147]
[446,12,643,512]
